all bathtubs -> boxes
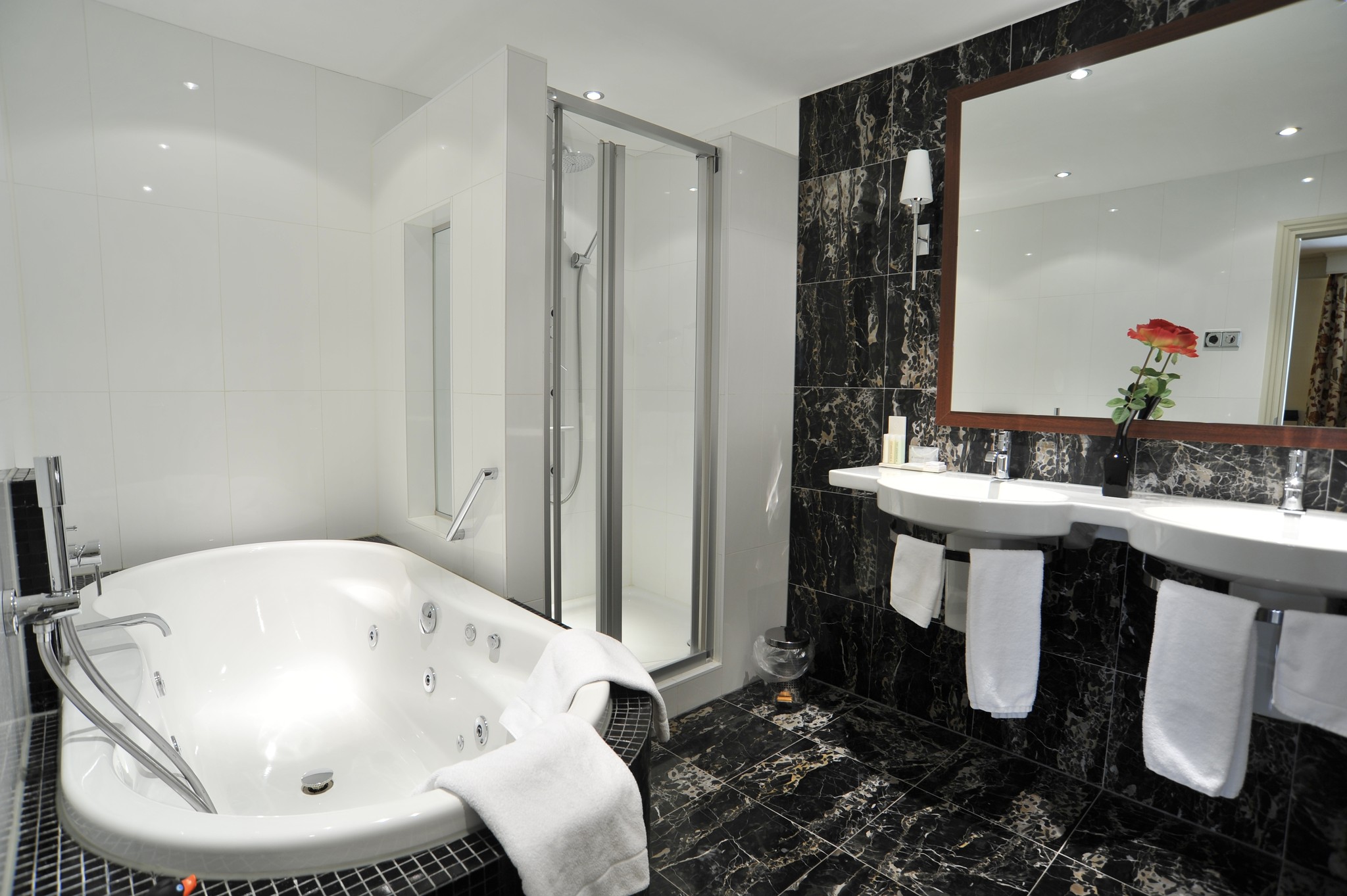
[49,539,615,876]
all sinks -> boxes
[1125,498,1347,597]
[875,467,1072,538]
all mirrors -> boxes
[935,0,1347,451]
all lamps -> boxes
[898,148,935,292]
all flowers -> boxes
[1104,316,1182,426]
[1137,325,1199,421]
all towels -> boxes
[1140,579,1260,800]
[499,630,672,741]
[412,711,652,896]
[965,547,1044,719]
[887,533,944,630]
[1268,610,1347,739]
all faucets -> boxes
[72,611,174,638]
[984,427,1013,481]
[1276,447,1307,511]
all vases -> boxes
[1099,383,1143,500]
[1138,392,1163,421]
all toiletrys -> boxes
[882,433,902,464]
